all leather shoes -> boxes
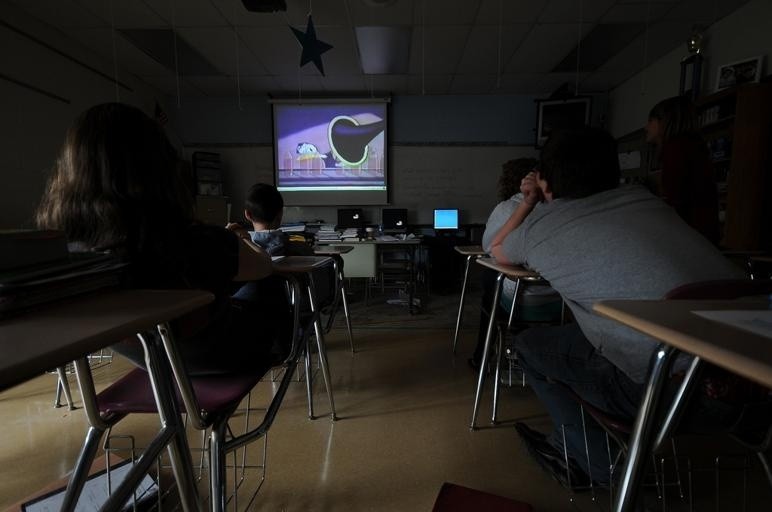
[468,358,481,370]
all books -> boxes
[701,105,729,222]
[0,230,128,317]
[192,152,225,196]
[281,224,362,242]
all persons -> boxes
[35,103,293,379]
[491,122,772,495]
[244,183,305,256]
[468,156,566,369]
[645,95,722,247]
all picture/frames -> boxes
[713,55,764,94]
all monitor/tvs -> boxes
[337,208,364,229]
[433,208,459,231]
[382,208,408,231]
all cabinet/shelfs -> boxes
[690,85,768,255]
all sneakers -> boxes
[512,420,590,492]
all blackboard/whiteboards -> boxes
[178,142,543,229]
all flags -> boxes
[155,102,170,125]
